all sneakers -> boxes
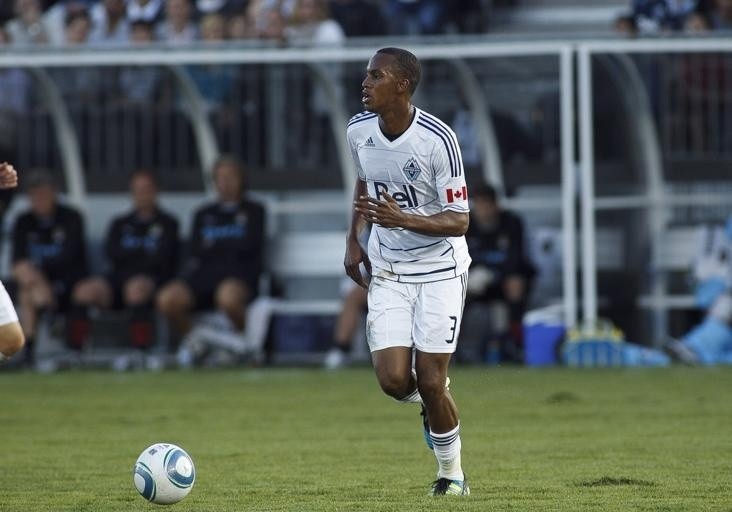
[428,472,471,497]
[420,376,450,450]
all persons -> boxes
[1,154,288,371]
[323,181,538,372]
[0,0,732,181]
[342,48,471,498]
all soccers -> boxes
[133,442,195,504]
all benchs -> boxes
[4,191,563,365]
